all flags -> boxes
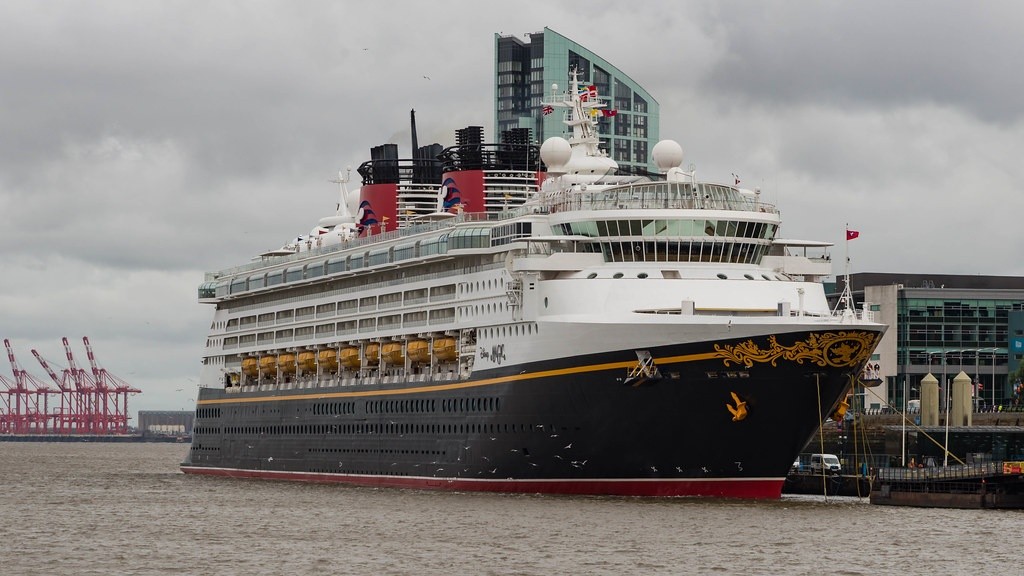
[736,179,740,184]
[543,106,554,116]
[846,230,859,240]
[578,85,596,101]
[589,108,618,117]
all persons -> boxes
[810,465,815,475]
[874,362,880,379]
[995,418,999,425]
[979,403,1003,413]
[866,363,873,379]
[907,454,927,468]
[1015,418,1020,426]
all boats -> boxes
[279,354,295,373]
[407,340,429,365]
[241,358,258,379]
[365,344,378,364]
[340,346,360,369]
[855,364,884,389]
[259,355,276,378]
[320,349,337,372]
[433,338,458,362]
[297,352,317,373]
[382,343,405,367]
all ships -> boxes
[173,64,889,506]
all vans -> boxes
[810,453,842,476]
[788,456,802,474]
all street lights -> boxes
[960,349,966,373]
[993,348,997,411]
[928,352,934,373]
[944,351,949,408]
[975,349,981,413]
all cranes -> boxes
[0,334,142,435]
